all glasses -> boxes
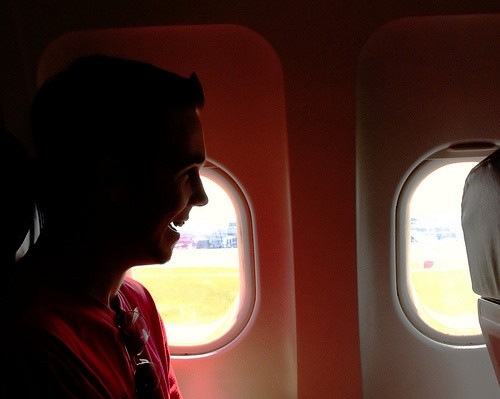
[112,307,159,396]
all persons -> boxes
[0,53,210,399]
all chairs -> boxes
[460,148,500,388]
[0,131,35,282]
